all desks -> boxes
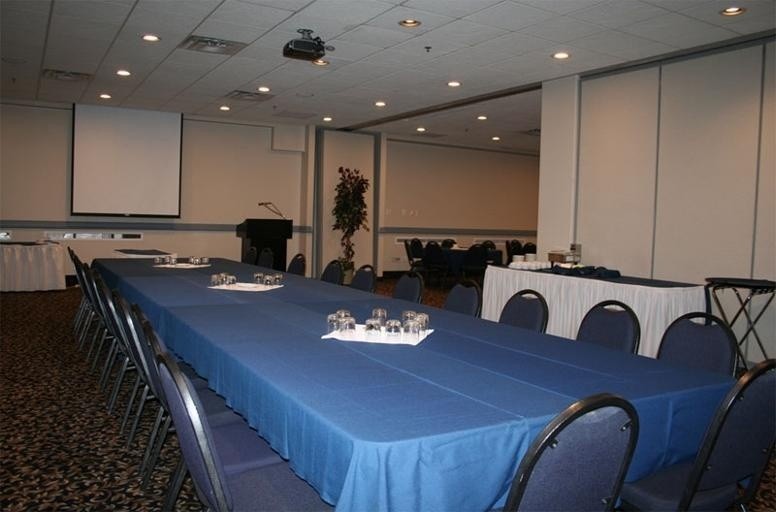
[481,264,705,360]
[706,278,776,370]
[92,258,753,512]
[0,242,66,291]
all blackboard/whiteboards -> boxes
[376,139,533,234]
[1,101,315,231]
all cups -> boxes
[253,271,283,289]
[154,256,177,266]
[508,253,551,270]
[209,272,237,289]
[188,255,209,266]
[327,306,430,343]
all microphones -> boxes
[259,202,283,216]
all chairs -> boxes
[499,289,548,334]
[444,280,482,318]
[503,394,638,512]
[576,300,640,356]
[404,238,536,291]
[621,358,776,512]
[350,265,377,292]
[392,271,425,304]
[657,312,739,379]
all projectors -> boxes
[281,38,325,62]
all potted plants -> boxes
[332,167,370,285]
